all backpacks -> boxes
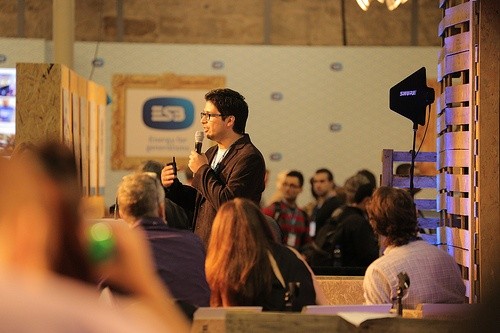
[300,213,365,275]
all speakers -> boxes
[388,67,427,126]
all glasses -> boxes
[201,113,228,121]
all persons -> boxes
[0,140,468,333]
[160,88,265,253]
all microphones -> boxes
[192,130,204,177]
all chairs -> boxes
[191,276,492,333]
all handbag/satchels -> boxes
[265,250,317,312]
